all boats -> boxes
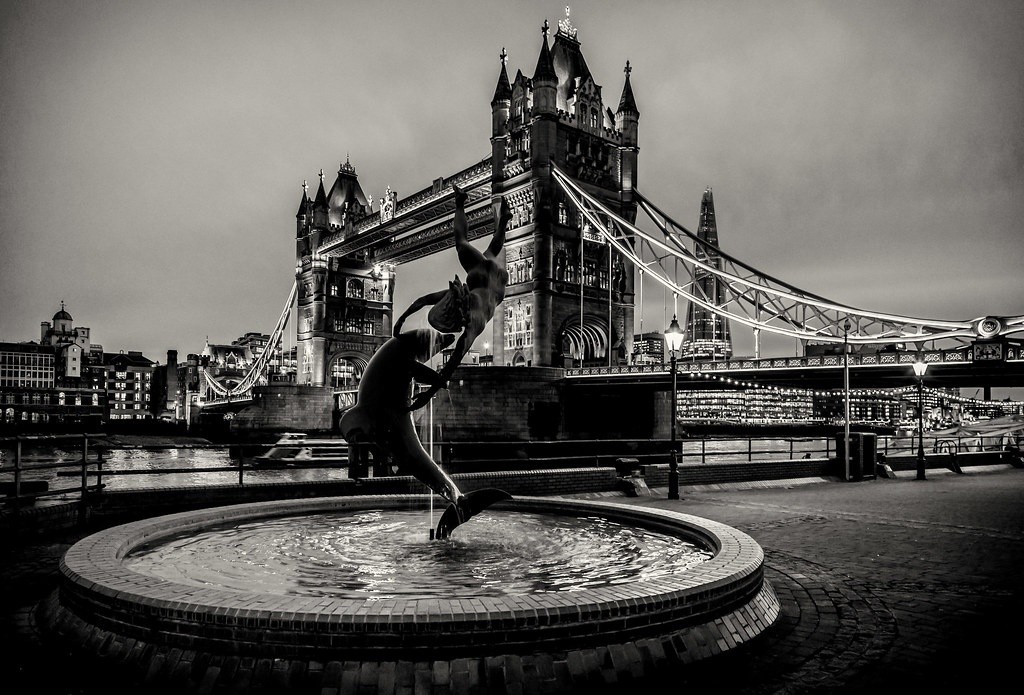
[251,431,394,468]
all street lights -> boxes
[844,318,852,482]
[663,313,687,501]
[911,351,929,481]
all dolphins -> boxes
[336,327,514,543]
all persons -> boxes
[392,186,514,411]
